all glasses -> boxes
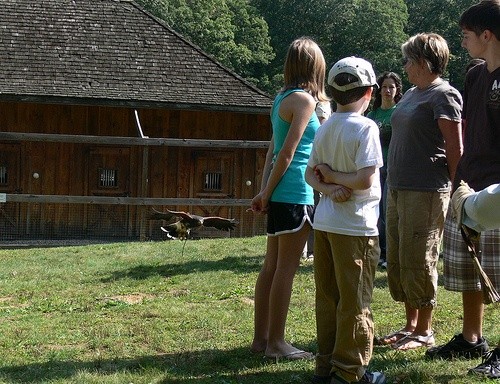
[401,57,415,64]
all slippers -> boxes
[265,351,312,361]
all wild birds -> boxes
[143,206,239,258]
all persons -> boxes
[380,33,463,350]
[427,1,500,384]
[252,40,320,359]
[306,56,384,382]
[367,72,402,269]
[448,181,496,233]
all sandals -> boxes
[384,330,412,344]
[389,331,435,350]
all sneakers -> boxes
[330,370,386,384]
[469,348,500,379]
[312,376,331,384]
[425,335,489,360]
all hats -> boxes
[328,56,380,91]
